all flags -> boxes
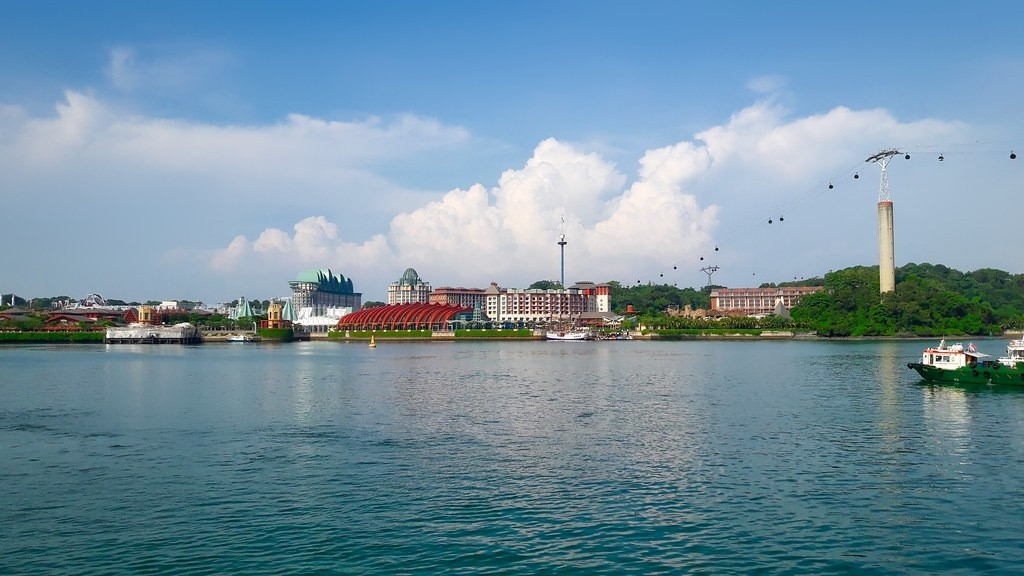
[970,343,977,352]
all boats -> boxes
[546,331,589,340]
[369,334,376,348]
[226,333,261,342]
[596,332,634,340]
[907,333,1024,386]
